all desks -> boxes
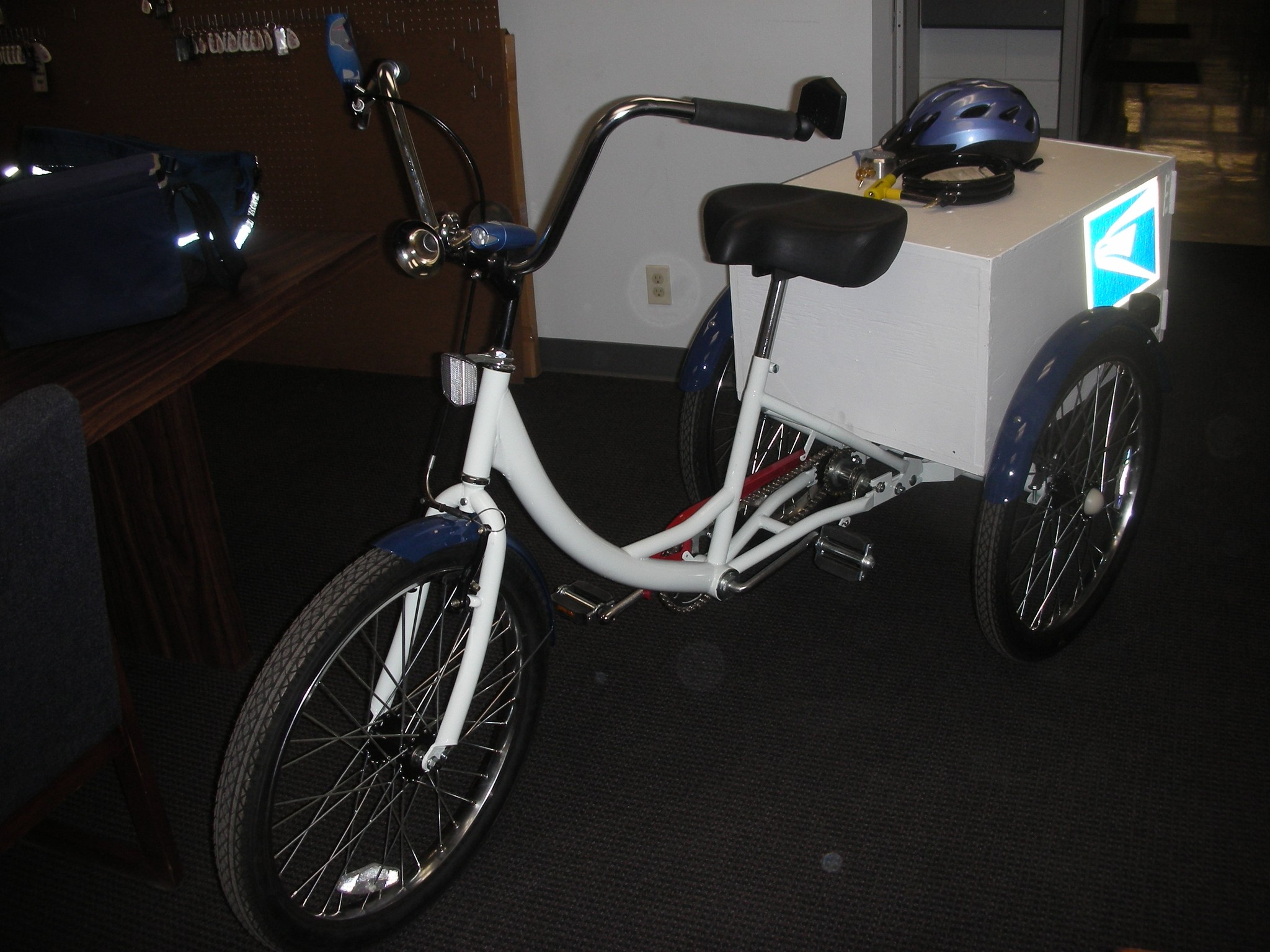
[0,232,378,672]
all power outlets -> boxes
[645,265,672,306]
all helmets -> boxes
[878,78,1041,166]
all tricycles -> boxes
[209,61,1179,952]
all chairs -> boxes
[0,383,182,889]
